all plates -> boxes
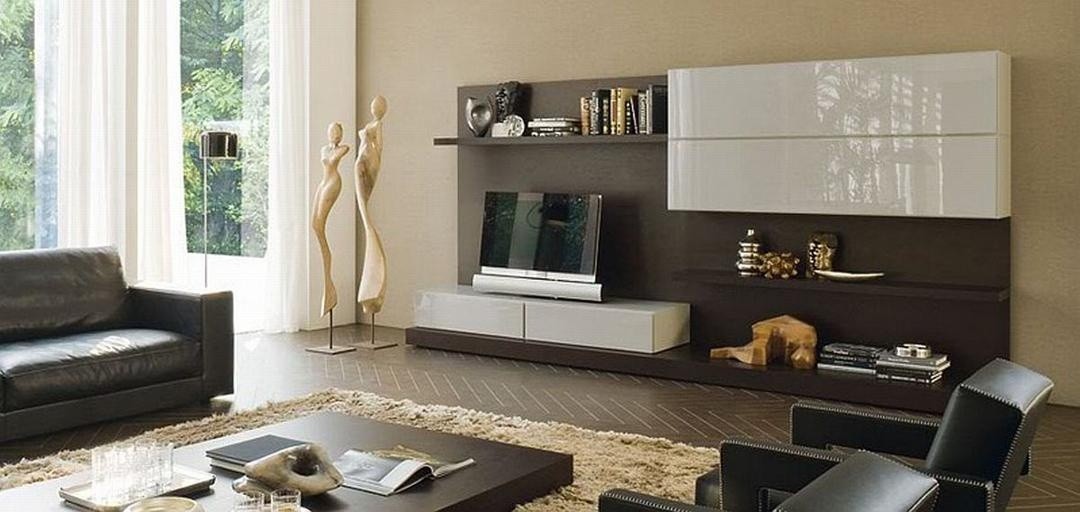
[504,114,526,139]
[230,502,311,510]
[814,267,884,283]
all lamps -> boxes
[197,127,239,287]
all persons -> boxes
[355,95,388,315]
[310,123,348,319]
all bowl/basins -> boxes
[124,495,207,511]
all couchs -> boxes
[0,244,239,447]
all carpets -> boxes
[2,382,721,510]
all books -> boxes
[526,115,583,138]
[330,444,478,499]
[204,433,312,475]
[578,81,669,136]
[816,338,952,386]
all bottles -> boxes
[735,228,766,278]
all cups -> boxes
[270,488,301,511]
[233,490,266,511]
[801,229,840,279]
[91,437,176,499]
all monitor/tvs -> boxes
[478,190,606,284]
